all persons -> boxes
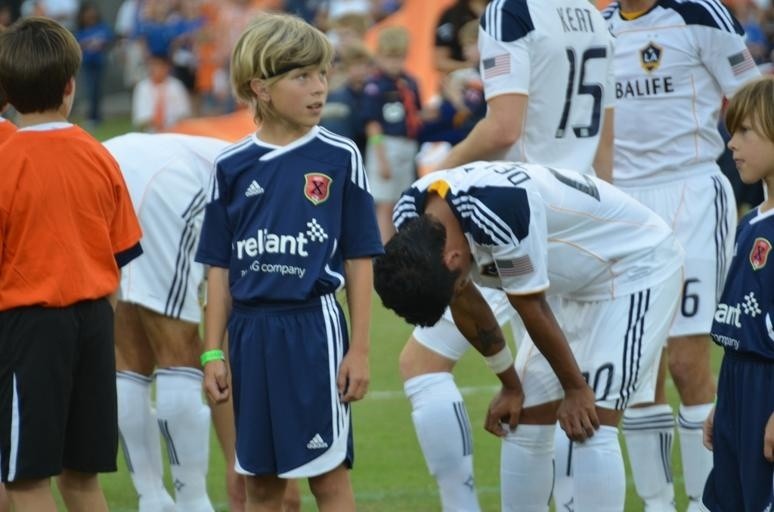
[0,0,774,511]
